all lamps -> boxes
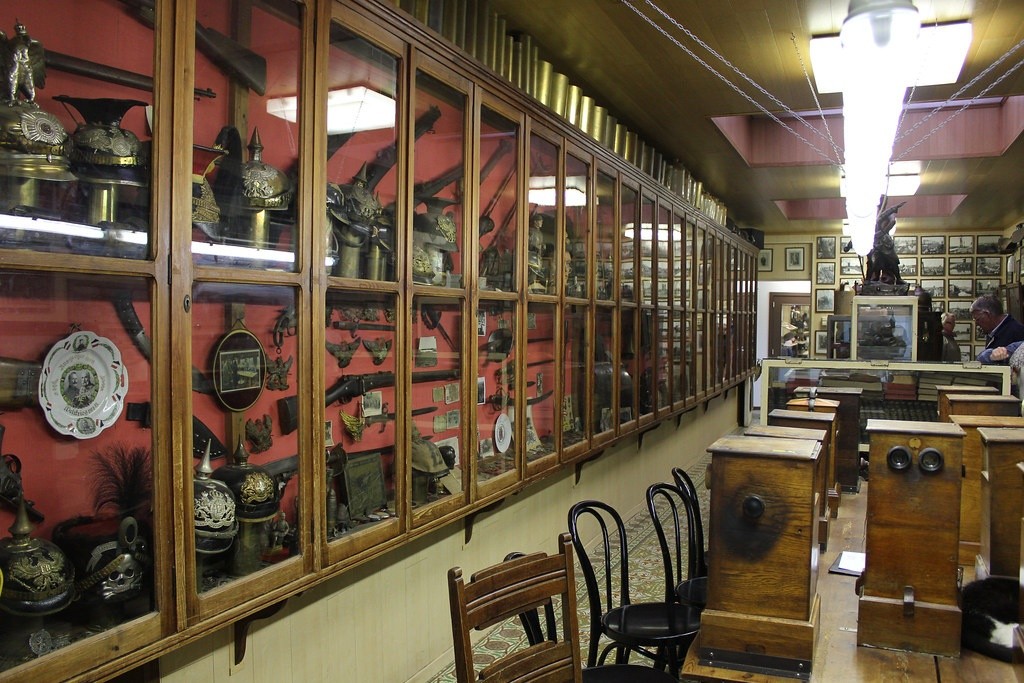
[811,0,974,255]
[625,223,682,242]
[528,174,600,207]
[266,85,397,137]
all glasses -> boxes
[973,313,983,323]
[946,321,955,327]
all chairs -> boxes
[446,466,708,683]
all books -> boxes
[828,550,866,578]
[884,370,1003,401]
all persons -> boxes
[529,214,546,283]
[63,370,95,401]
[843,194,908,285]
[969,294,1024,399]
[975,341,1024,417]
[546,242,572,295]
[941,312,961,362]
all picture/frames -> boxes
[841,237,857,254]
[757,248,773,271]
[817,261,835,285]
[815,330,828,354]
[839,257,864,275]
[817,236,836,259]
[839,278,863,295]
[892,234,1024,362]
[785,247,804,271]
[816,289,835,313]
